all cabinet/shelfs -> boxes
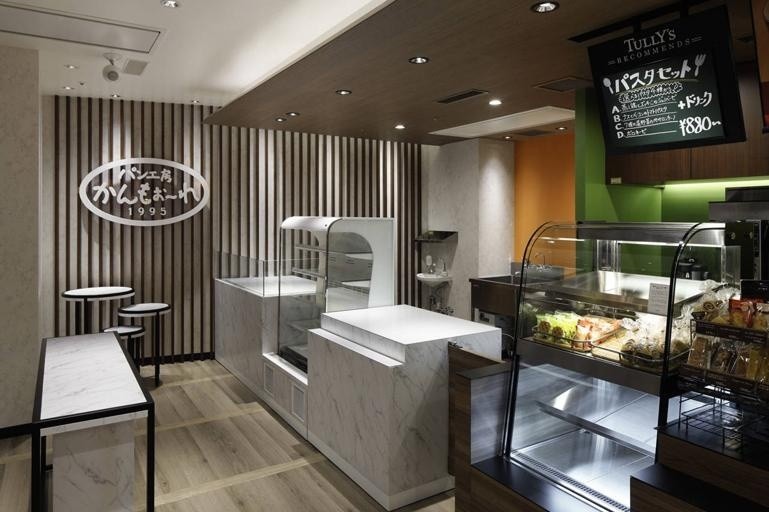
[276,216,396,372]
[605,35,769,186]
[502,218,760,511]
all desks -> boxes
[30,330,155,512]
[61,285,137,333]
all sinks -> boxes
[468,275,552,317]
[415,272,450,287]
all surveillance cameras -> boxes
[102,64,121,84]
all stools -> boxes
[101,325,146,375]
[117,302,172,386]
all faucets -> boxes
[427,267,435,275]
[514,270,522,278]
